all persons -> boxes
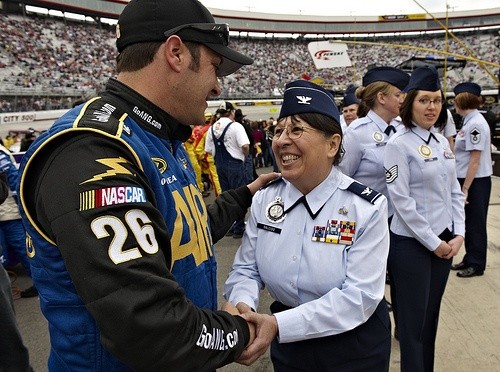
[334,66,411,340]
[222,80,391,372]
[16,0,282,372]
[452,82,493,278]
[0,83,496,372]
[0,11,500,110]
[384,66,465,372]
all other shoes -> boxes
[226,229,233,236]
[233,228,245,239]
[456,267,485,277]
[451,260,467,270]
[20,286,39,298]
[386,301,393,311]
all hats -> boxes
[235,108,247,121]
[453,82,481,96]
[219,101,237,113]
[344,83,356,96]
[276,80,340,124]
[342,94,359,107]
[361,66,411,91]
[405,64,441,92]
[115,0,254,77]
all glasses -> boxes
[419,98,442,105]
[266,126,325,141]
